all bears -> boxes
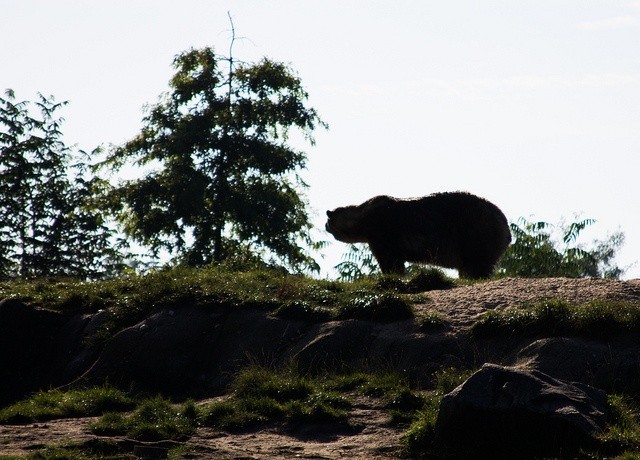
[325,190,514,281]
